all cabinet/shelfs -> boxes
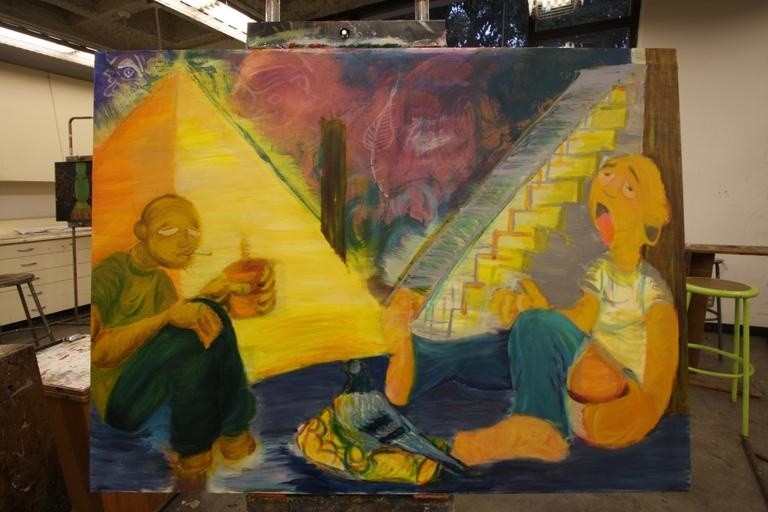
[0,344,71,512]
[0,236,91,327]
[36,333,179,512]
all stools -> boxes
[686,275,759,438]
[0,273,55,347]
[704,259,723,362]
